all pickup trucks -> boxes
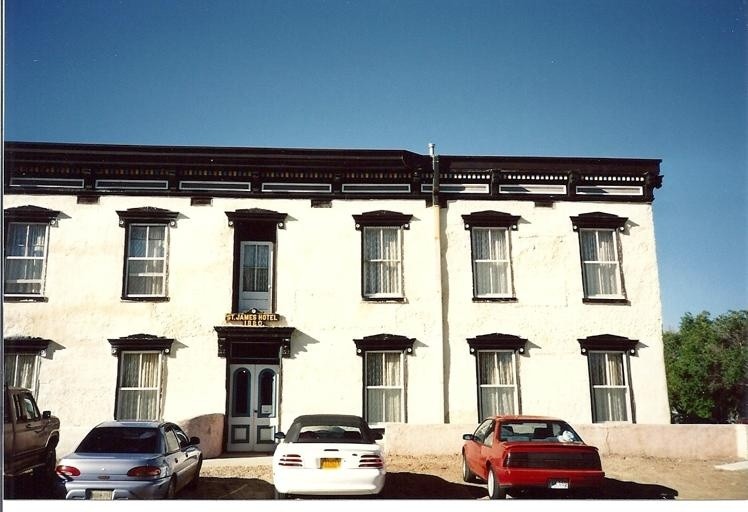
[3,383,61,488]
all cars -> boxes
[455,415,606,498]
[48,417,204,497]
[267,415,386,500]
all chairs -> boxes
[300,430,319,438]
[533,427,552,438]
[501,425,514,435]
[342,430,360,440]
